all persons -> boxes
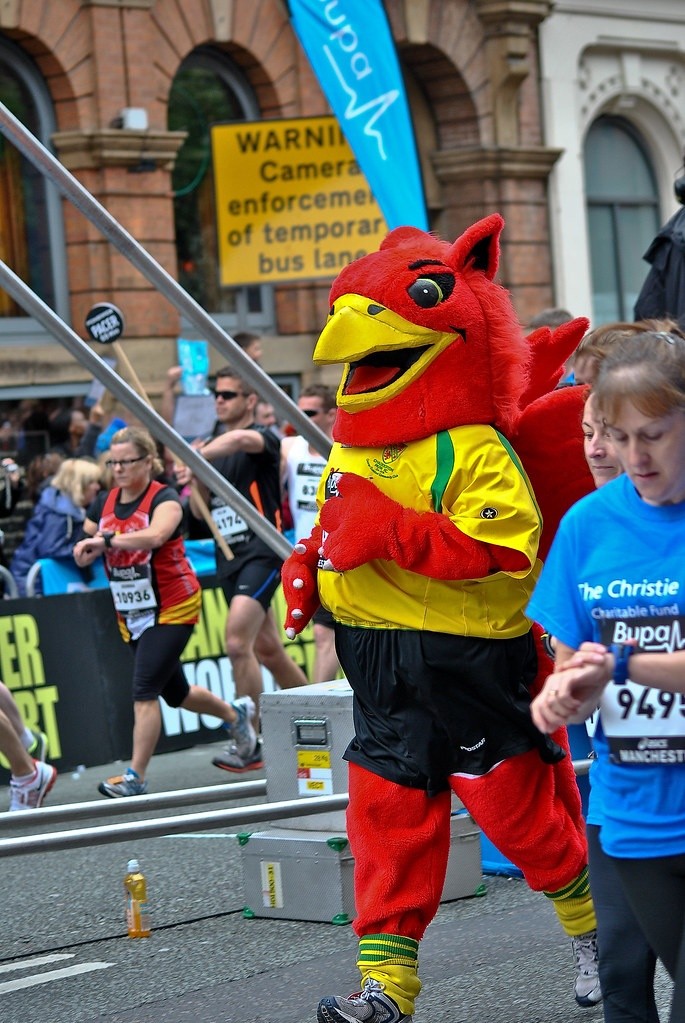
[573,319,685,386]
[0,332,281,519]
[524,332,685,1023]
[540,389,626,655]
[73,425,256,798]
[0,680,57,812]
[7,458,104,598]
[173,365,310,773]
[280,382,338,684]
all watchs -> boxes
[102,530,115,548]
[607,642,646,685]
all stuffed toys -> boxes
[282,213,603,1023]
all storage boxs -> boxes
[236,811,487,926]
[258,679,465,832]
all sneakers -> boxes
[98,767,148,798]
[8,731,57,812]
[316,978,414,1023]
[231,697,256,760]
[212,739,264,772]
[571,929,602,1007]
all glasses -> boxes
[215,390,249,400]
[105,453,149,469]
[305,410,317,417]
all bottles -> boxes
[124,858,150,938]
